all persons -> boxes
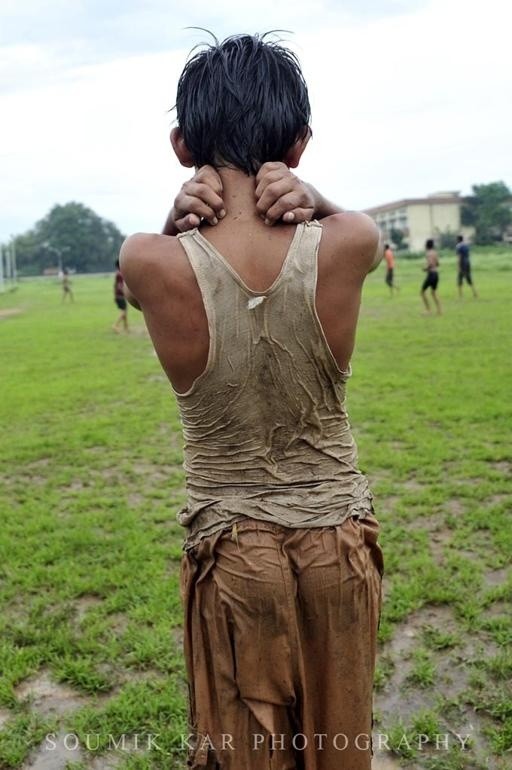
[383,244,402,300]
[418,238,444,317]
[112,257,132,338]
[453,234,479,299]
[61,268,77,305]
[118,34,387,769]
